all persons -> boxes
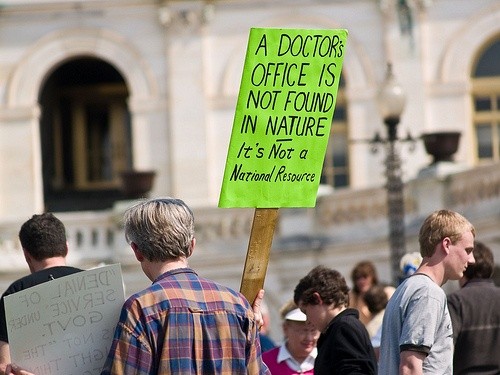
[0,199,500,375]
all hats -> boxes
[284,307,307,321]
[398,252,423,278]
